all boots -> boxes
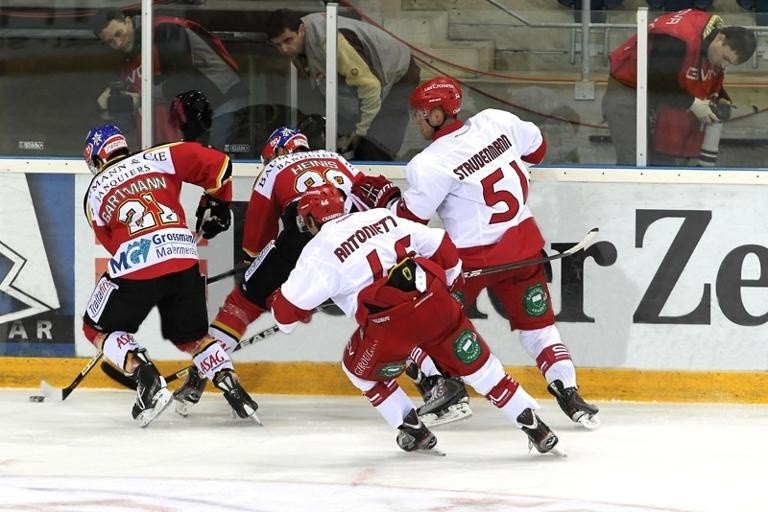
[393,407,438,452]
[130,350,168,418]
[409,371,471,417]
[544,377,599,422]
[515,407,560,454]
[210,367,259,418]
[170,365,208,405]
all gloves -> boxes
[195,194,233,240]
[351,174,403,214]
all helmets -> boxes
[407,73,462,119]
[81,123,129,164]
[258,124,310,167]
[297,184,344,221]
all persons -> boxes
[601,8,756,166]
[267,183,560,453]
[81,122,259,423]
[167,89,324,159]
[390,76,599,424]
[265,8,420,161]
[91,6,249,155]
[469,75,580,163]
[174,127,467,415]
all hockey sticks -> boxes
[41,217,213,400]
[101,324,279,391]
[462,228,599,277]
